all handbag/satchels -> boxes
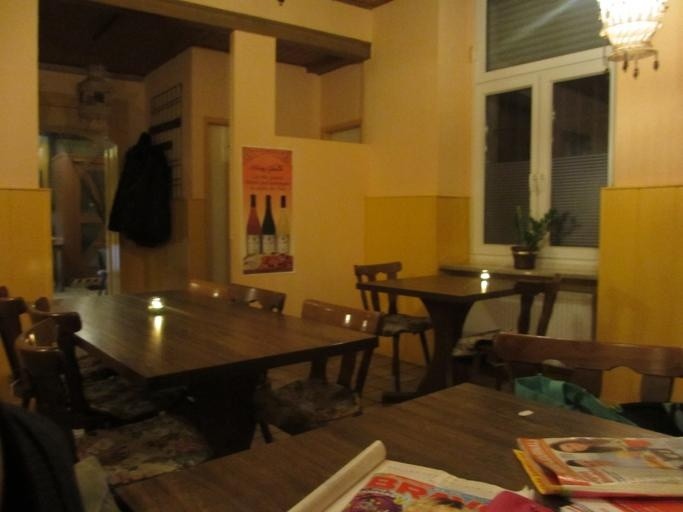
[192,368,263,455]
[514,373,641,429]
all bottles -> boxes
[277,195,289,254]
[247,195,260,254]
[260,195,276,255]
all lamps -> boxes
[596,0,667,77]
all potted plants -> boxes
[511,206,558,269]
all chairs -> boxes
[229,281,287,313]
[1,285,165,459]
[256,299,385,444]
[460,272,562,392]
[184,277,234,304]
[354,262,434,395]
[492,331,681,407]
[1,400,87,510]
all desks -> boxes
[30,283,382,454]
[104,380,680,512]
[356,274,545,394]
